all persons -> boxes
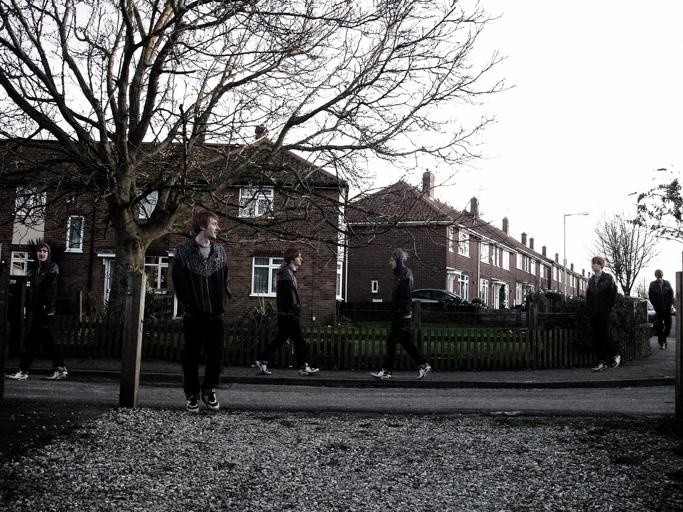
[3,240,69,381]
[171,209,231,413]
[648,269,674,349]
[581,256,621,373]
[368,248,432,380]
[250,247,319,377]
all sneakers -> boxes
[186,397,199,412]
[253,358,271,374]
[45,366,68,379]
[4,370,29,381]
[592,360,608,371]
[201,392,219,410]
[611,355,621,369]
[416,363,431,379]
[299,362,319,376]
[369,367,391,379]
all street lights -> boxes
[563,212,590,303]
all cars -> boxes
[638,299,664,334]
[411,288,470,307]
